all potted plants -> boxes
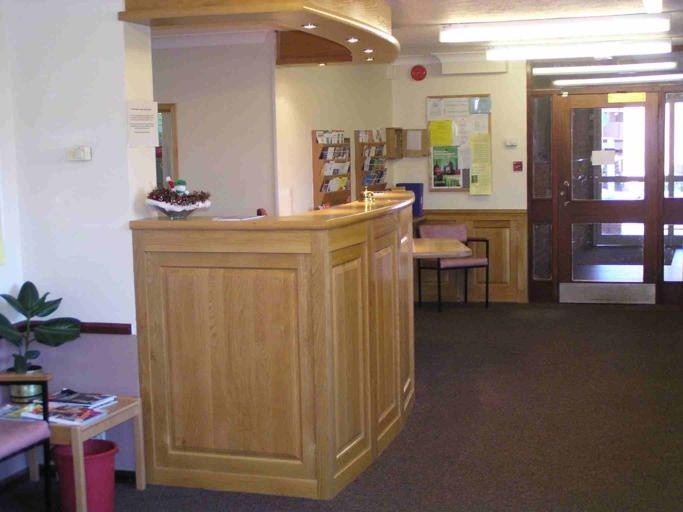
[0,280,81,405]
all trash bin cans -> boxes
[49,438,121,512]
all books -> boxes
[20,388,118,426]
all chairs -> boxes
[417,224,488,312]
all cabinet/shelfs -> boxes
[311,130,387,210]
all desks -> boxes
[1,389,146,512]
